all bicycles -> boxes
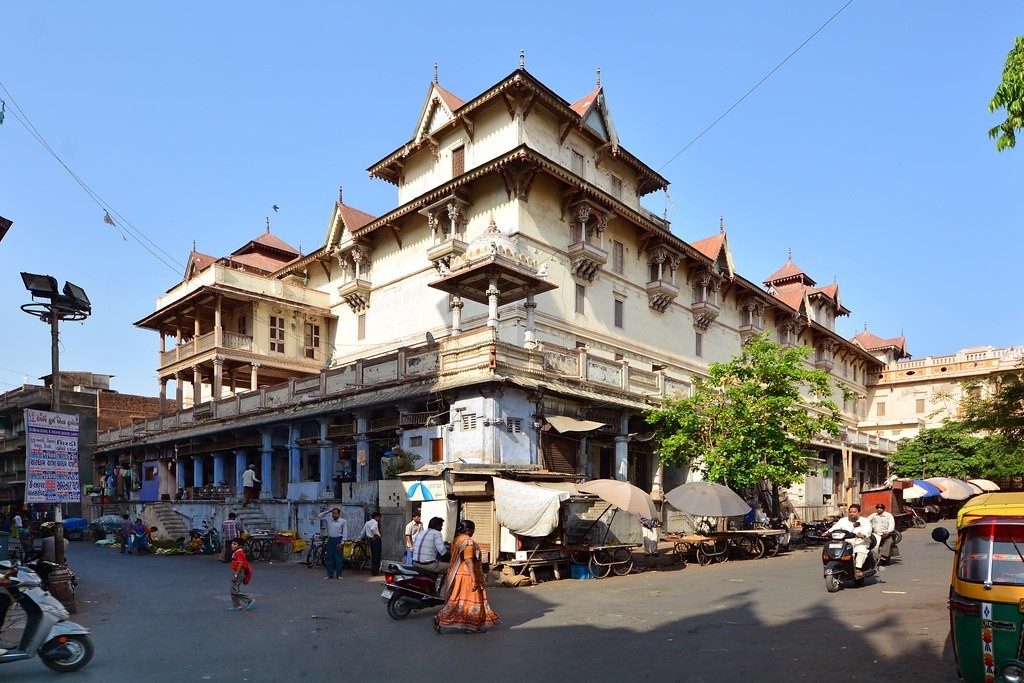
[350,540,372,572]
[304,533,328,568]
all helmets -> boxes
[875,504,884,509]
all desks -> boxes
[498,557,577,586]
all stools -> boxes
[791,519,801,529]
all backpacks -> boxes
[243,564,253,585]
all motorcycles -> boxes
[931,492,1024,683]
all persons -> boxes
[412,517,449,596]
[120,514,158,555]
[0,509,69,583]
[356,512,382,576]
[867,504,895,561]
[221,512,255,611]
[242,464,261,507]
[318,508,347,580]
[405,512,424,564]
[822,504,872,577]
[186,532,202,553]
[918,497,926,503]
[641,517,659,558]
[433,519,503,634]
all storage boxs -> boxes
[272,529,307,553]
[571,564,600,579]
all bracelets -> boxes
[864,534,866,538]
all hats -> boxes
[249,464,255,469]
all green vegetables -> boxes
[147,540,160,553]
[169,546,177,554]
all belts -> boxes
[413,561,434,565]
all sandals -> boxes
[465,627,486,634]
[433,615,442,635]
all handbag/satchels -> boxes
[352,542,361,559]
[342,541,352,558]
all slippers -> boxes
[855,570,862,577]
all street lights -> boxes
[20,273,91,600]
[921,453,928,481]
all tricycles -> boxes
[241,530,275,560]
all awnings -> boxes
[540,412,613,447]
[491,475,596,575]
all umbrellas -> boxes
[903,476,1000,499]
[577,479,657,545]
[663,481,753,531]
[90,514,124,525]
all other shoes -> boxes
[246,599,255,611]
[0,649,8,655]
[0,637,17,649]
[227,606,241,611]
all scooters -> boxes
[759,517,790,551]
[800,521,834,545]
[871,531,902,565]
[818,522,880,592]
[28,553,77,598]
[381,563,444,621]
[0,550,95,673]
[189,521,220,554]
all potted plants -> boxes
[378,445,424,508]
